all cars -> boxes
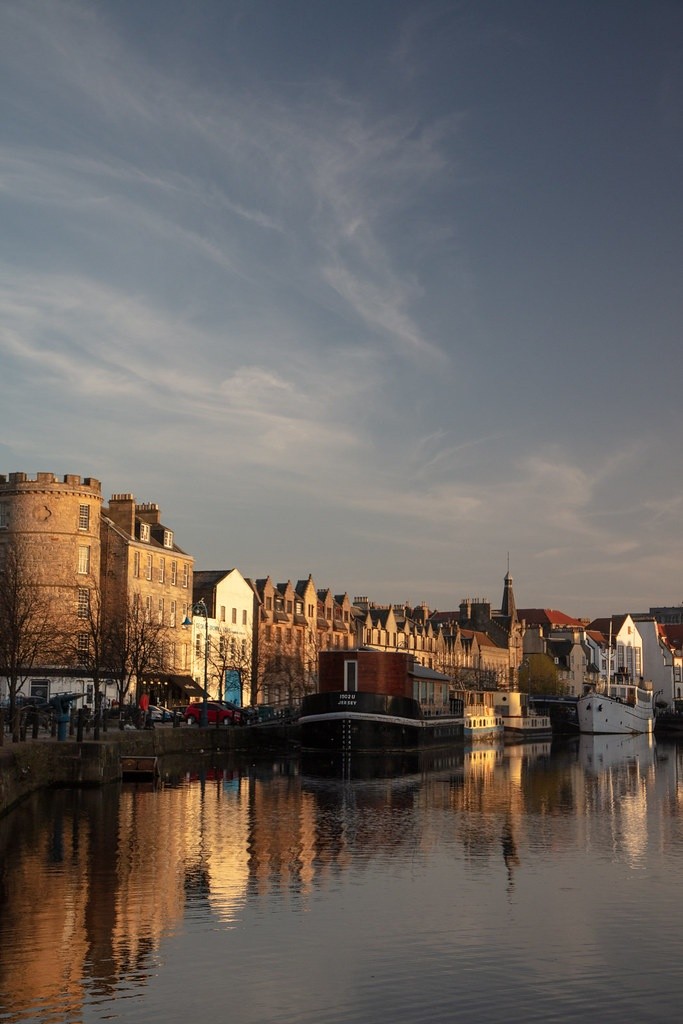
[148,701,255,727]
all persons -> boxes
[112,698,119,709]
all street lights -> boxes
[182,602,209,728]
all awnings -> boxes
[167,674,211,699]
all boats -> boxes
[577,683,657,734]
[298,644,466,752]
